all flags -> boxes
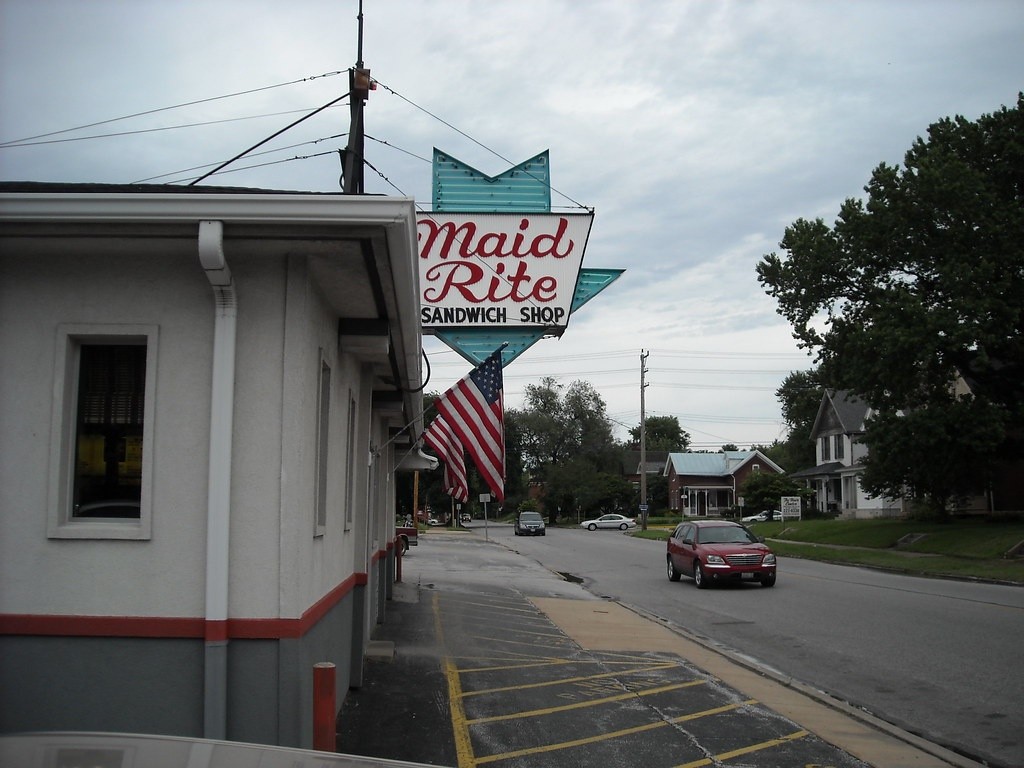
[421,413,468,504]
[431,344,506,508]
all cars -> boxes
[462,513,472,522]
[581,513,636,530]
[741,509,782,523]
[667,519,777,589]
[428,517,438,524]
[514,511,545,536]
